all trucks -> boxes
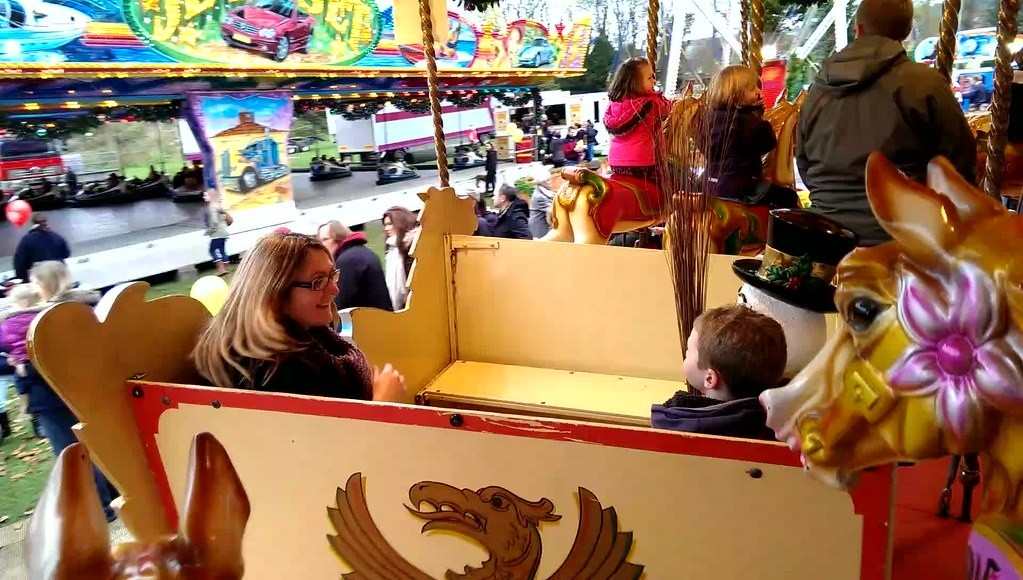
[333,92,519,164]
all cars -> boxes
[518,37,557,66]
[221,0,314,64]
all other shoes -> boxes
[34,421,45,437]
[0,413,10,437]
[104,509,117,528]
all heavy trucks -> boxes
[0,129,70,212]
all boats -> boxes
[399,43,459,65]
[0,0,93,55]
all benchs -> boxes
[125,240,864,580]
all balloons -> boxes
[5,199,32,228]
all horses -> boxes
[534,88,806,256]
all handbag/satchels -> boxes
[218,212,233,226]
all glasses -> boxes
[290,269,342,291]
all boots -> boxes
[213,260,224,275]
[223,262,230,273]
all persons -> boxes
[954,75,990,112]
[491,182,533,240]
[694,66,803,208]
[14,260,121,523]
[317,220,394,312]
[13,212,71,283]
[651,305,787,442]
[472,191,498,236]
[795,0,977,240]
[527,169,557,239]
[484,142,498,193]
[521,113,599,168]
[312,148,483,170]
[382,206,420,310]
[188,232,408,403]
[38,165,204,195]
[603,56,700,192]
[203,188,230,276]
[0,283,55,376]
